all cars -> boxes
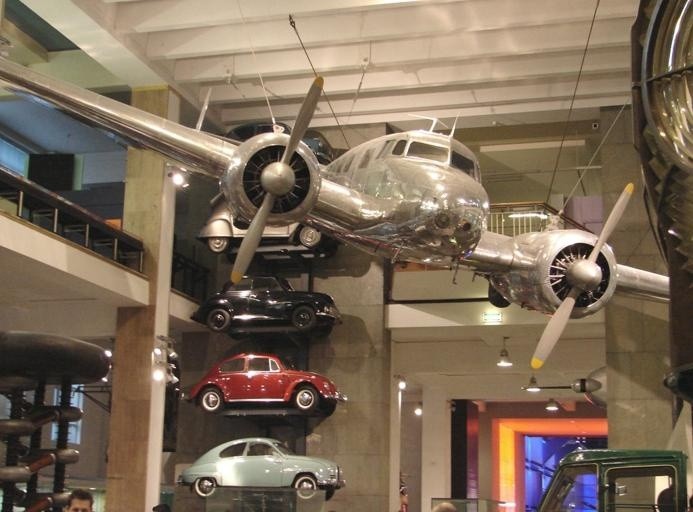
[189,276,342,340]
[185,352,348,420]
[200,190,340,267]
[177,436,346,500]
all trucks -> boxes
[533,447,689,512]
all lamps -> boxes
[494,339,559,412]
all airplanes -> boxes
[0,59,670,369]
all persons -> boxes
[65,489,93,512]
[432,502,456,512]
[400,472,409,512]
